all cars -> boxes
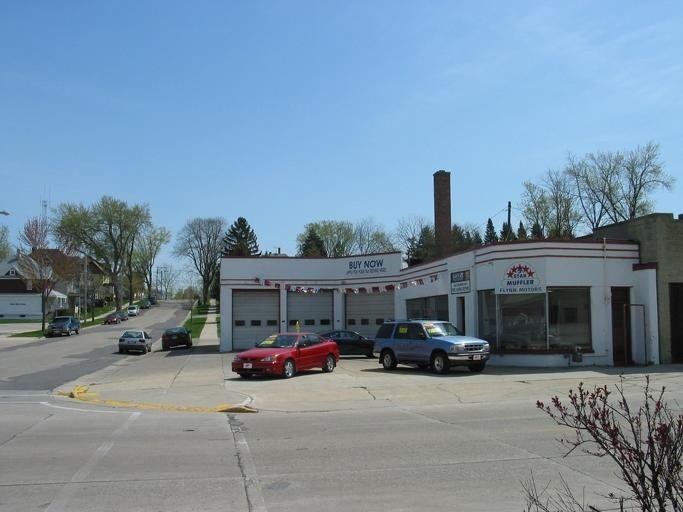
[162,327,192,350]
[318,329,381,358]
[232,332,339,378]
[119,331,152,353]
[103,301,151,324]
[48,316,80,336]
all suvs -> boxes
[373,318,490,374]
